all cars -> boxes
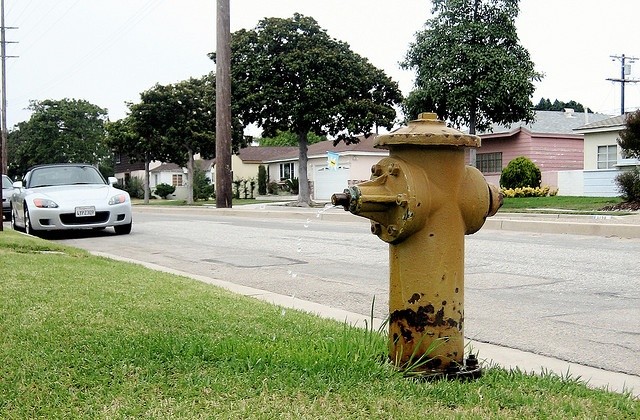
[14,163,132,236]
[2,175,21,221]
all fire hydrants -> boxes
[331,112,504,383]
